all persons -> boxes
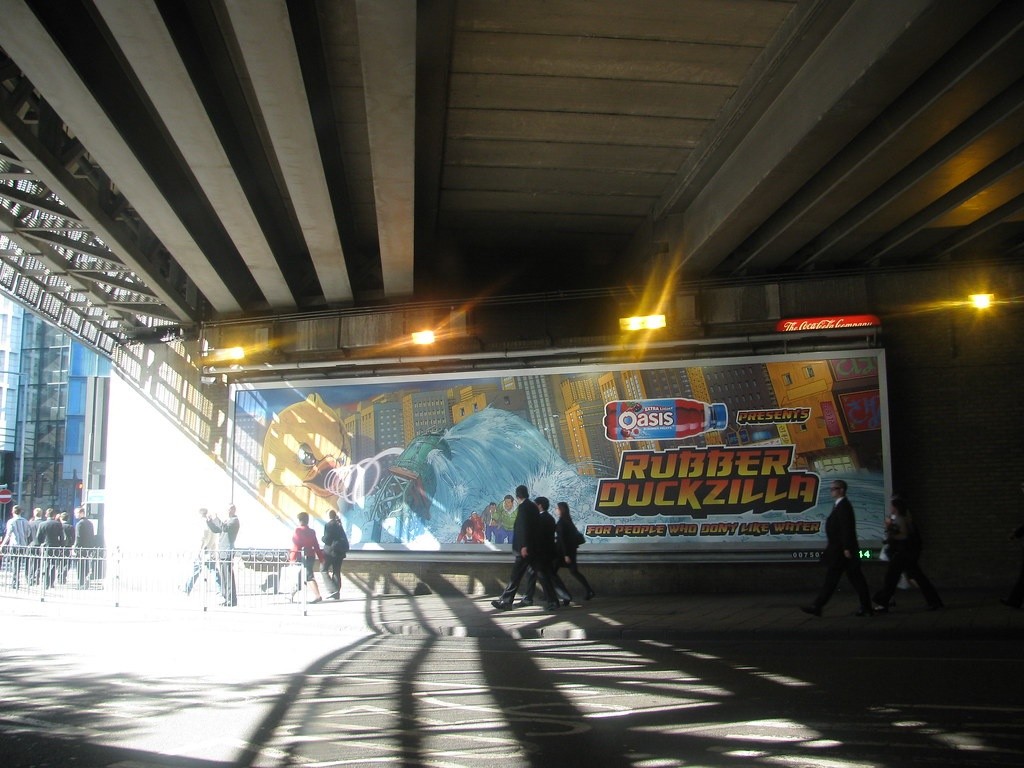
[317,508,349,602]
[178,507,231,600]
[867,498,945,614]
[281,510,322,605]
[544,502,597,600]
[71,505,97,590]
[490,486,561,611]
[203,504,242,608]
[516,497,575,606]
[797,477,881,618]
[996,478,1024,612]
[0,504,32,593]
[879,495,917,605]
[29,506,74,593]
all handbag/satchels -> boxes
[280,567,302,592]
[577,533,585,545]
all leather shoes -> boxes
[491,601,512,611]
[515,601,533,607]
[854,607,876,617]
[544,603,560,610]
[538,596,546,601]
[584,591,595,600]
[563,595,572,607]
[800,606,822,618]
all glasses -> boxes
[831,486,844,491]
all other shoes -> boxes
[326,590,340,600]
[873,605,888,614]
[926,602,943,611]
[11,575,89,589]
[306,596,322,604]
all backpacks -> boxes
[326,523,348,559]
